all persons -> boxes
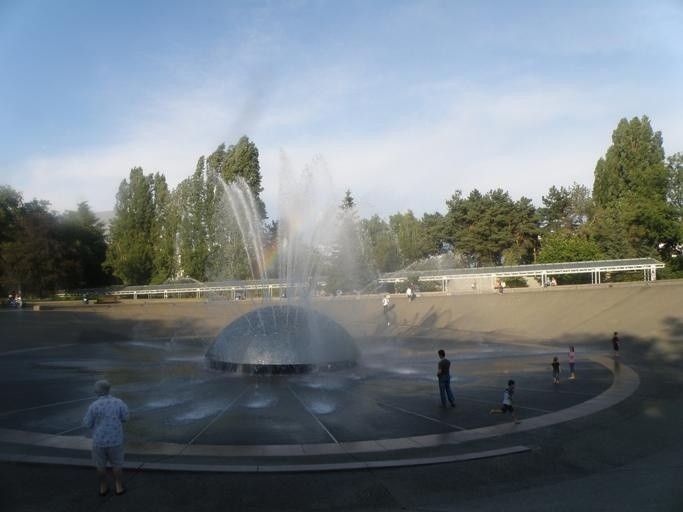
[384,291,390,307]
[381,294,389,313]
[409,283,419,298]
[435,349,457,407]
[488,379,522,424]
[495,279,500,293]
[549,276,557,286]
[551,356,562,383]
[610,331,621,358]
[405,285,412,303]
[543,277,550,287]
[81,378,129,497]
[565,345,576,380]
[499,279,505,293]
